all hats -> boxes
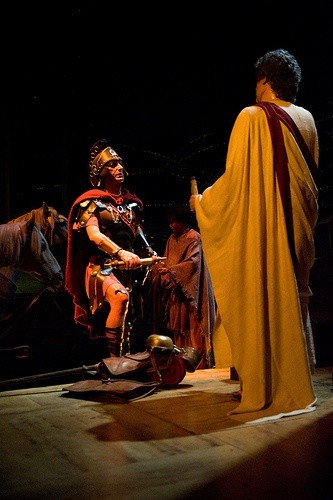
[88,139,128,187]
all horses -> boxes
[0,202,68,328]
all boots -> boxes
[105,327,122,358]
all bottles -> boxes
[189,176,200,212]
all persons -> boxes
[147,207,216,370]
[64,139,161,358]
[189,49,319,424]
